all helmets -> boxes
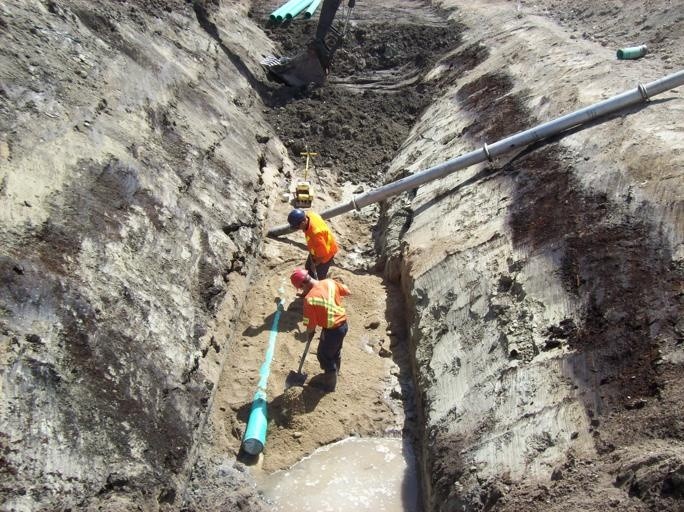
[289,267,309,289]
[286,209,307,231]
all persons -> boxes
[289,268,353,389]
[286,209,341,298]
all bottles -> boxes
[616,44,648,58]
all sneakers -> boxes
[296,290,308,298]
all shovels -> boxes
[286,333,314,388]
